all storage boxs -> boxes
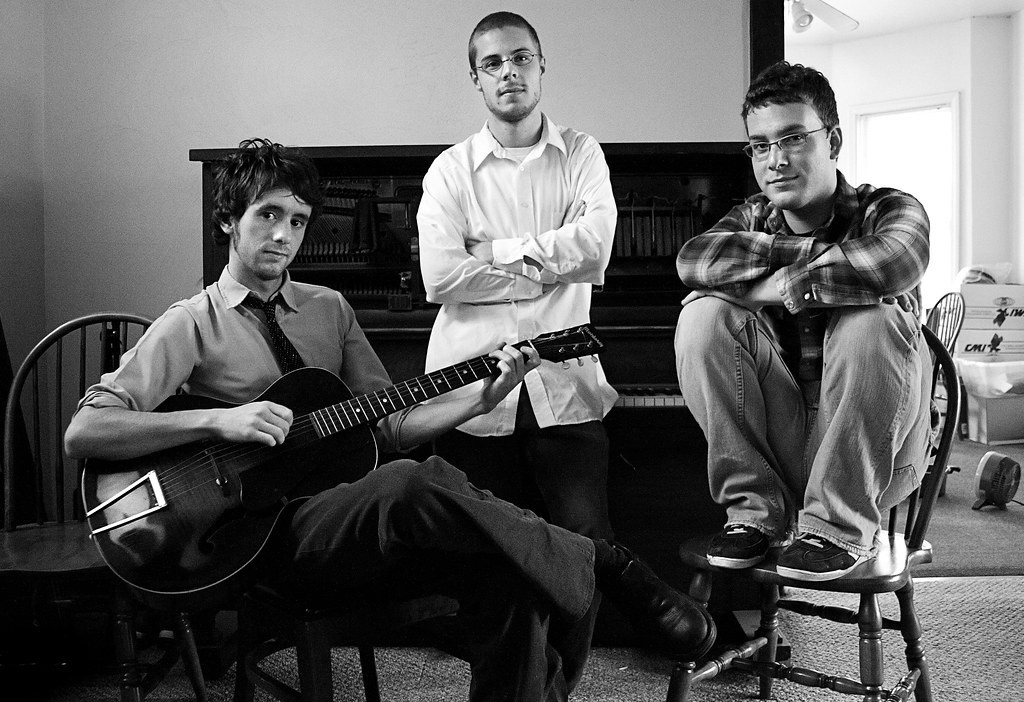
[954,259,1024,445]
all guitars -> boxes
[82,322,608,594]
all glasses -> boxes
[742,127,826,158]
[473,51,542,73]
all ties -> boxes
[240,295,310,376]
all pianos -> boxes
[186,137,792,663]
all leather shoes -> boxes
[615,545,716,664]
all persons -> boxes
[61,136,719,702]
[670,60,937,588]
[414,4,619,690]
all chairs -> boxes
[923,292,966,376]
[663,317,963,702]
[0,311,210,701]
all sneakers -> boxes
[775,533,880,582]
[705,525,794,569]
